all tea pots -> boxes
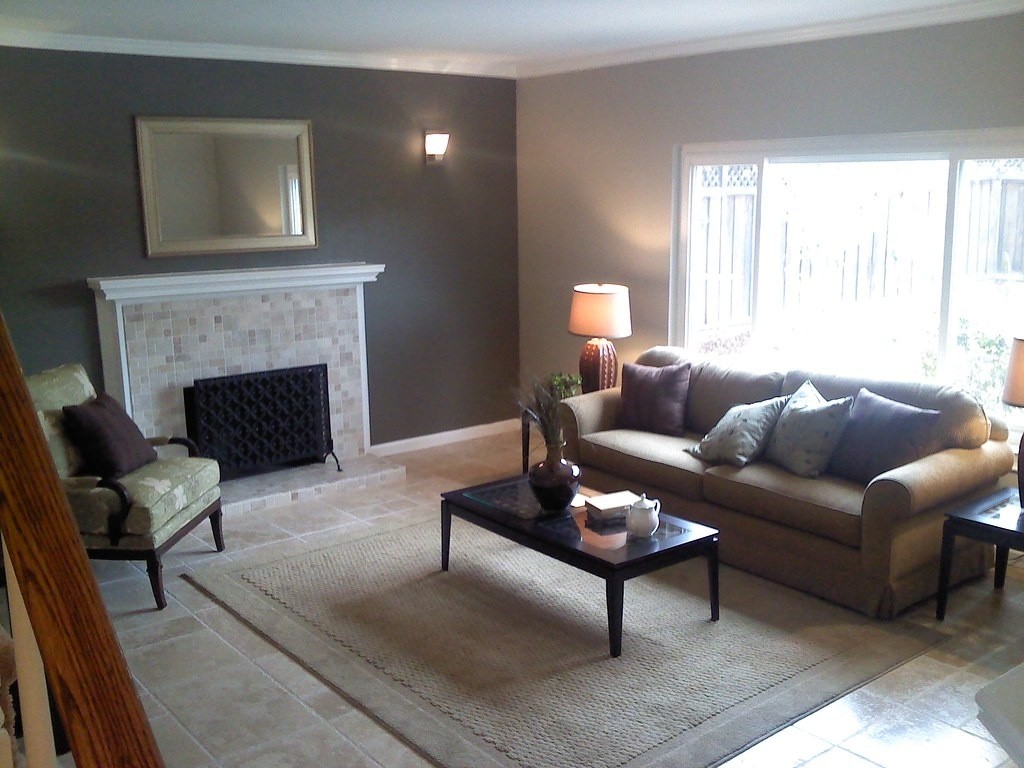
[624,493,661,538]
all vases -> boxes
[526,440,583,513]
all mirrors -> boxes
[135,114,319,260]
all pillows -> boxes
[822,388,941,487]
[682,393,796,470]
[763,378,854,481]
[59,394,159,487]
[620,361,694,439]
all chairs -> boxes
[25,362,228,611]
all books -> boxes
[585,490,642,520]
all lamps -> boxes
[1001,338,1024,509]
[423,130,452,172]
[567,281,632,395]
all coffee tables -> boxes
[437,476,719,660]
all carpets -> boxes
[181,509,953,768]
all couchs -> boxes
[555,344,1014,622]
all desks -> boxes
[522,404,567,478]
[934,486,1024,622]
